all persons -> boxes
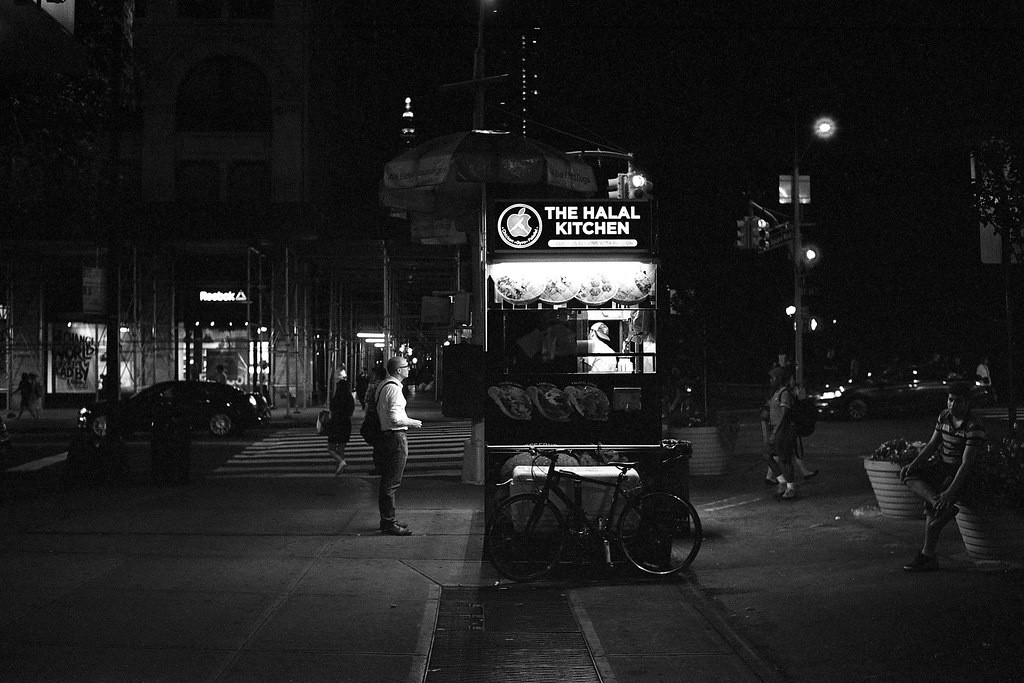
[668,373,697,427]
[215,364,225,384]
[325,379,355,475]
[580,321,617,373]
[897,383,987,571]
[759,368,819,501]
[355,362,387,408]
[374,355,422,537]
[413,361,420,392]
[28,368,46,416]
[976,355,998,406]
[925,352,941,367]
[10,372,37,420]
[953,354,964,368]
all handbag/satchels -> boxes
[360,411,381,444]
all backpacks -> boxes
[779,389,818,437]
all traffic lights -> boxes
[627,172,647,201]
[749,218,769,252]
[737,217,750,251]
[608,173,626,199]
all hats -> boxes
[590,322,610,341]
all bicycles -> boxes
[483,448,703,583]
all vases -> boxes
[953,483,1024,560]
[864,457,927,521]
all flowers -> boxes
[867,436,927,464]
[979,438,1024,500]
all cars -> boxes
[808,360,987,423]
[77,380,270,441]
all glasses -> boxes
[396,364,410,370]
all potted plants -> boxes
[666,397,741,476]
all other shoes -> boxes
[381,525,412,536]
[929,504,960,529]
[764,478,781,486]
[775,496,798,502]
[379,518,408,530]
[774,491,786,498]
[904,553,928,572]
[337,460,348,473]
[804,469,819,480]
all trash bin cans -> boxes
[662,439,693,537]
[153,434,191,487]
